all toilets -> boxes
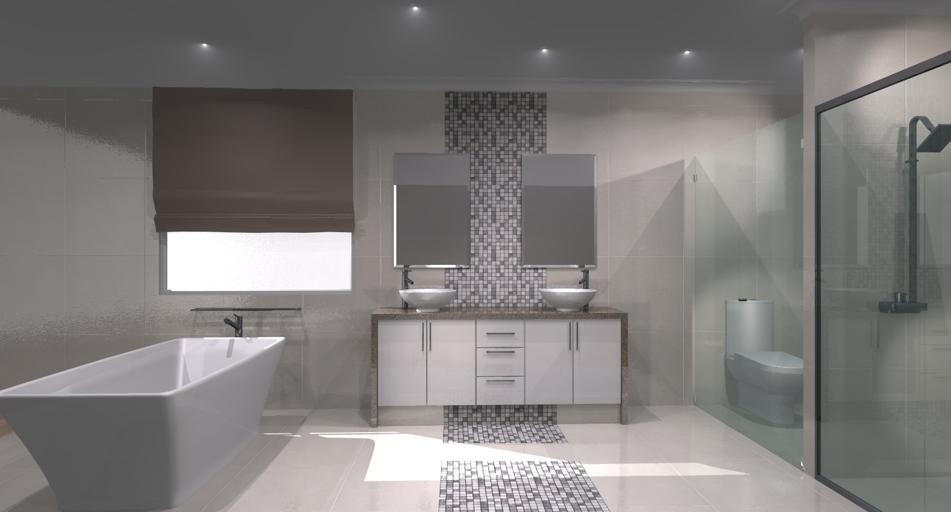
[725,298,803,425]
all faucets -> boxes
[224,313,242,337]
[402,264,413,309]
[579,270,589,308]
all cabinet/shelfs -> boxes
[378,319,476,407]
[525,318,622,405]
[476,318,525,405]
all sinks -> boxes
[399,288,457,312]
[539,288,598,312]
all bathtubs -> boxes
[0,336,286,511]
